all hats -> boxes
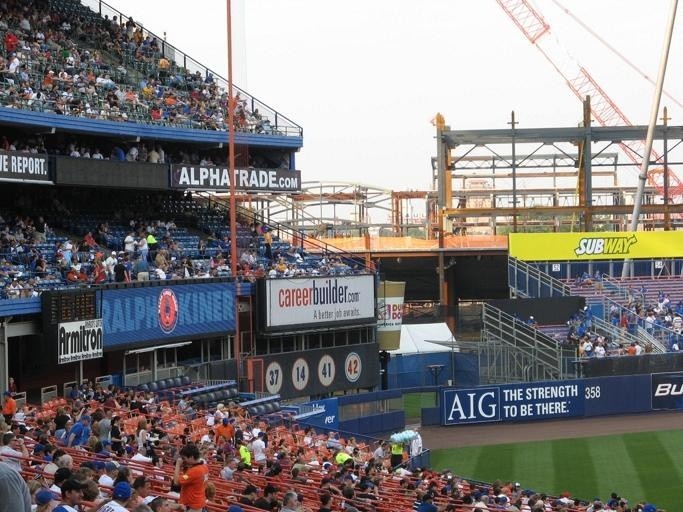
[214,400,308,462]
[51,380,157,455]
[54,468,73,483]
[60,480,89,492]
[112,482,136,499]
[2,391,12,395]
[35,490,54,506]
[227,505,243,512]
[67,249,178,274]
[33,444,46,453]
[263,484,279,494]
[321,462,589,508]
[104,460,121,472]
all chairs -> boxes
[0,367,298,512]
[1,0,292,169]
[299,420,652,512]
[1,181,363,301]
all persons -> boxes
[509,270,682,365]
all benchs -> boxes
[529,268,683,358]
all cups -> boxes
[370,279,406,351]
